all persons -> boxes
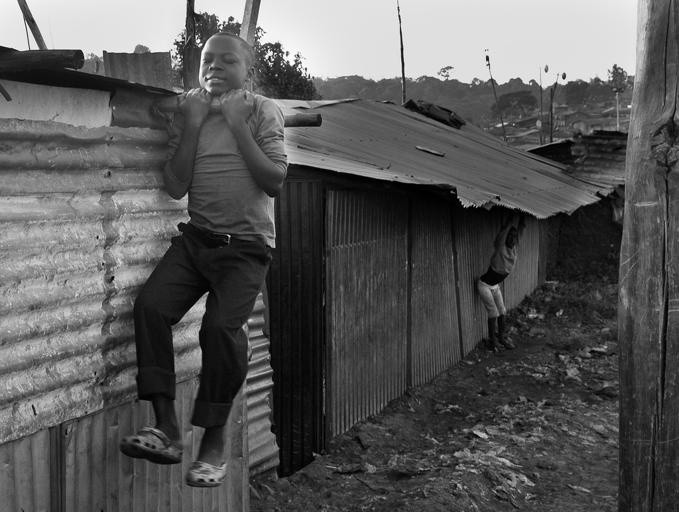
[477,211,528,349]
[119,32,289,487]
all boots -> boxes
[487,315,516,353]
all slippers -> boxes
[119,426,229,488]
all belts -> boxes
[177,222,264,249]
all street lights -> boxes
[543,62,566,141]
[612,86,626,130]
